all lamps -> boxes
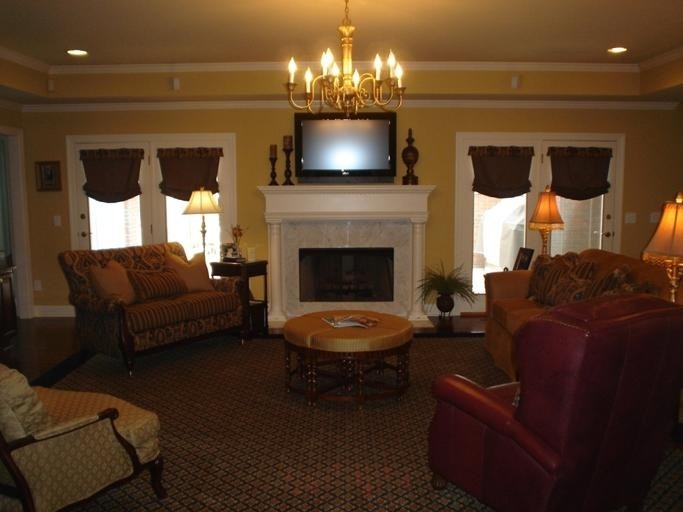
[640,187,683,301]
[528,181,564,254]
[282,0,408,117]
[181,183,221,253]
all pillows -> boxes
[122,265,188,302]
[163,248,218,292]
[524,252,649,311]
[84,257,136,305]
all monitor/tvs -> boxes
[294,111,397,184]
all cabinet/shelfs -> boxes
[0,264,23,364]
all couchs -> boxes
[0,359,166,511]
[59,242,248,376]
[483,245,683,385]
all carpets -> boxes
[0,327,509,511]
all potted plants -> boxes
[414,259,475,322]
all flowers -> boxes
[224,222,249,253]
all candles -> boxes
[282,135,293,149]
[268,143,277,159]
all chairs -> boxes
[427,297,683,512]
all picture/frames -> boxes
[34,159,61,193]
[512,247,533,270]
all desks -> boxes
[279,311,414,409]
[208,258,272,336]
